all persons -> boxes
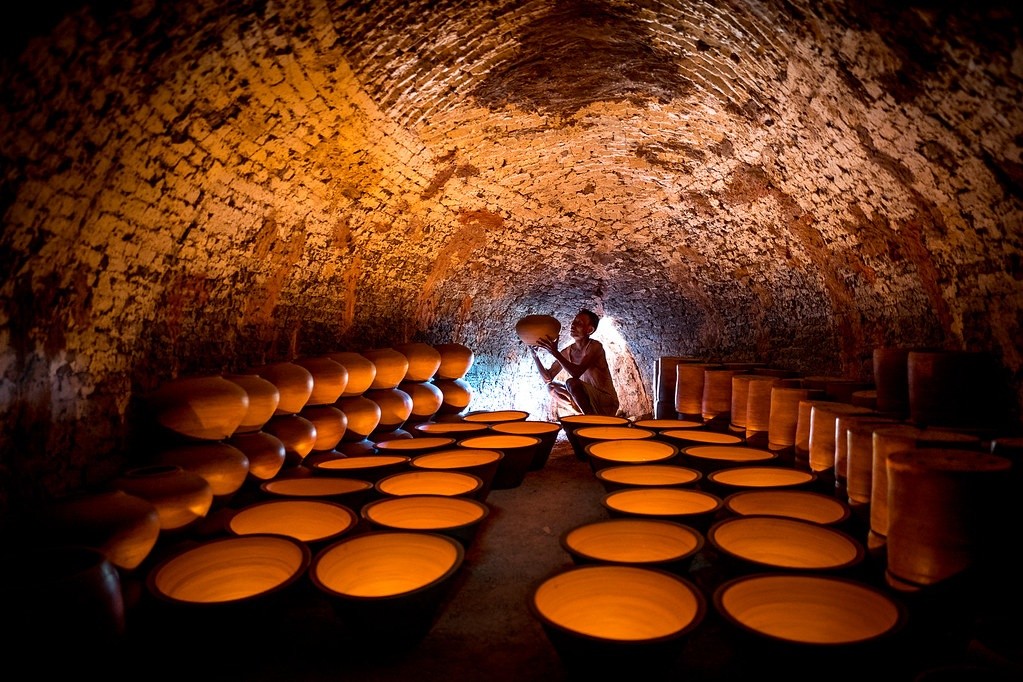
[527,310,619,417]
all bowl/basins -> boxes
[223,498,359,551]
[456,434,543,490]
[373,470,489,503]
[308,529,466,640]
[723,489,852,526]
[558,415,631,459]
[585,438,679,471]
[489,421,563,470]
[372,436,456,456]
[146,532,311,635]
[312,454,411,484]
[601,486,723,533]
[680,445,779,492]
[460,410,529,425]
[659,430,746,465]
[409,448,506,488]
[260,477,373,508]
[708,465,819,494]
[573,427,656,458]
[712,571,908,682]
[595,464,703,490]
[365,493,489,551]
[526,566,706,677]
[414,424,490,440]
[560,518,706,574]
[632,420,706,442]
[708,514,864,578]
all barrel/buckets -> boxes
[654,346,1022,652]
